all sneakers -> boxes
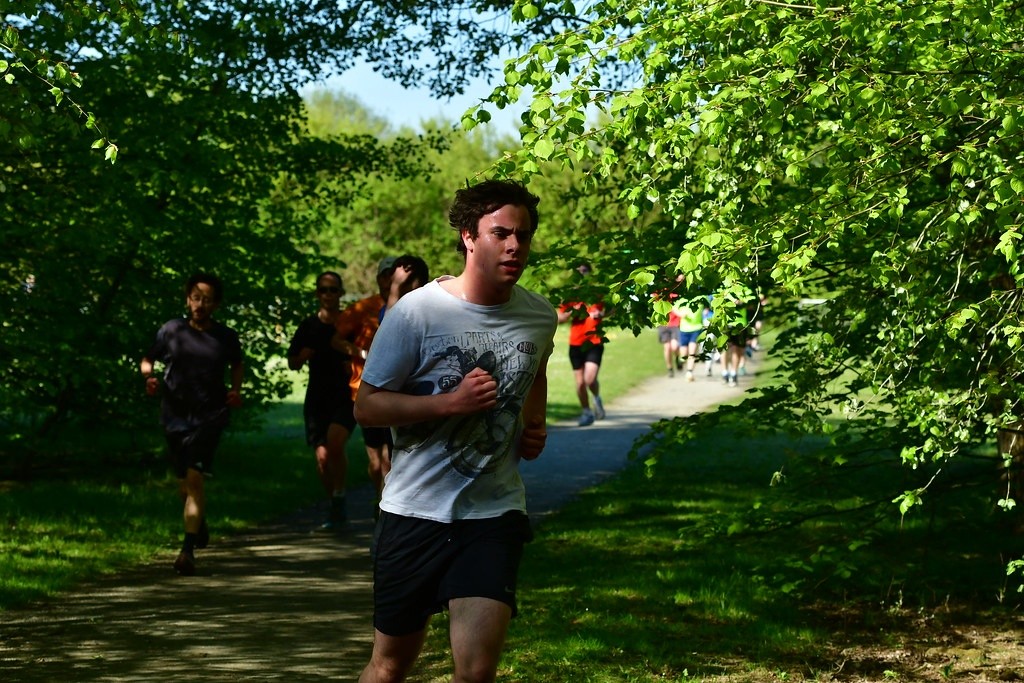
[594,406,605,420]
[729,374,739,387]
[737,366,746,376]
[174,551,196,576]
[318,513,352,530]
[578,412,594,427]
[194,516,210,549]
[675,356,683,371]
[719,370,731,385]
[685,371,695,381]
[668,369,675,378]
[704,367,712,377]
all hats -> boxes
[376,255,400,275]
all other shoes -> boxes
[750,339,759,352]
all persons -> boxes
[374,251,431,328]
[648,256,788,386]
[287,269,363,535]
[332,256,423,503]
[137,272,247,574]
[554,257,611,434]
[352,176,560,682]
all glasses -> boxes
[317,285,342,294]
